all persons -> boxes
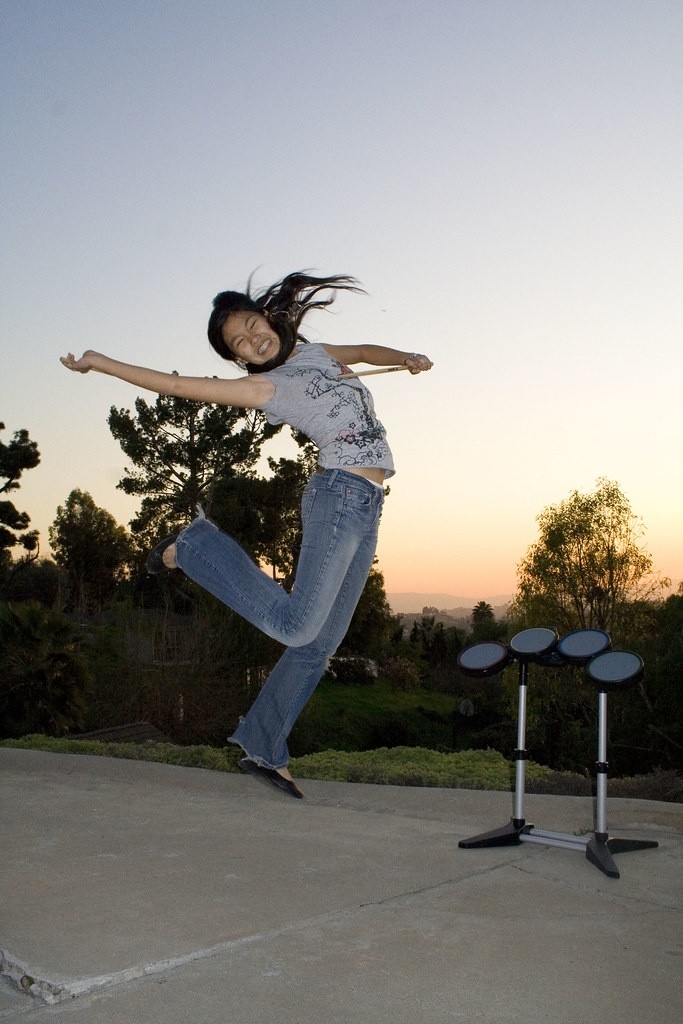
[59,270,434,801]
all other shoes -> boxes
[238,751,304,799]
[146,533,180,574]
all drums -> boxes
[558,630,611,666]
[457,640,510,679]
[509,627,557,661]
[587,649,643,690]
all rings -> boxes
[410,353,421,359]
[404,359,410,366]
